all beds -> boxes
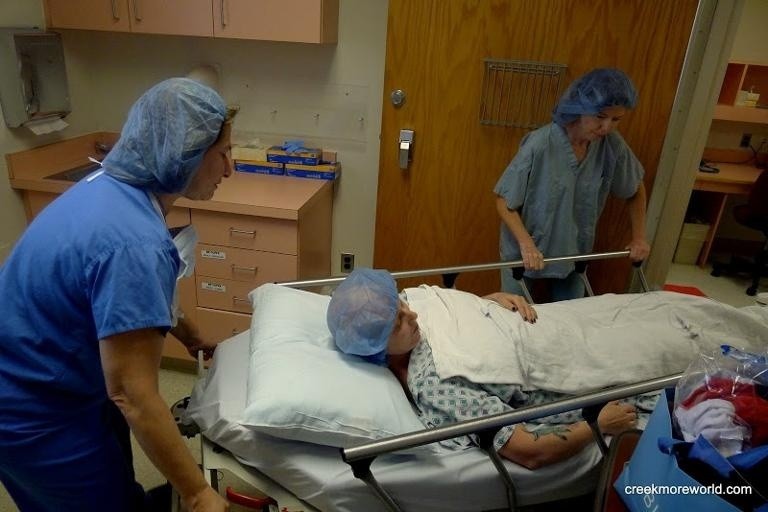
[165,248,768,512]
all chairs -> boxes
[711,165,768,295]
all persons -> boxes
[319,262,664,471]
[492,64,651,305]
[1,76,236,512]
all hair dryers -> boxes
[230,137,272,162]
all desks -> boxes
[691,163,766,270]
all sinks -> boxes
[94,143,113,155]
[42,159,104,182]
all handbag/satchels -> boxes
[613,386,767,512]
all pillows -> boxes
[237,282,428,450]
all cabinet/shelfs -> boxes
[22,186,336,368]
[212,0,339,45]
[41,1,214,38]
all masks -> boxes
[170,223,195,280]
